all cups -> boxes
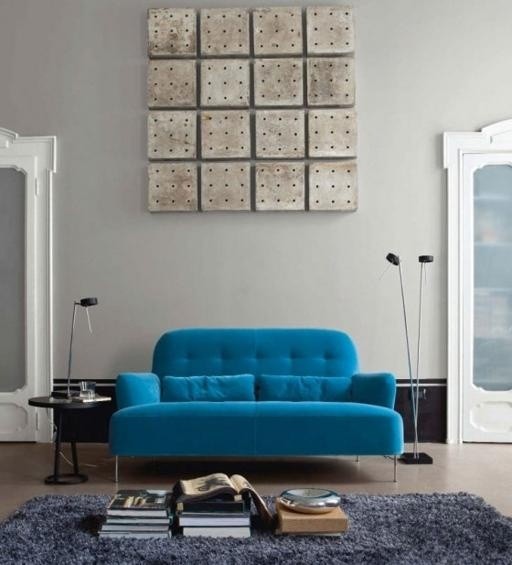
[78,380,96,402]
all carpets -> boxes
[0,493,512,565]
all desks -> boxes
[29,396,112,485]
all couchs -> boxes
[108,328,404,483]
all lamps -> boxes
[386,254,433,464]
[52,297,97,397]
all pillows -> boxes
[162,374,255,402]
[258,374,352,402]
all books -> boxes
[71,393,112,403]
[98,473,274,539]
